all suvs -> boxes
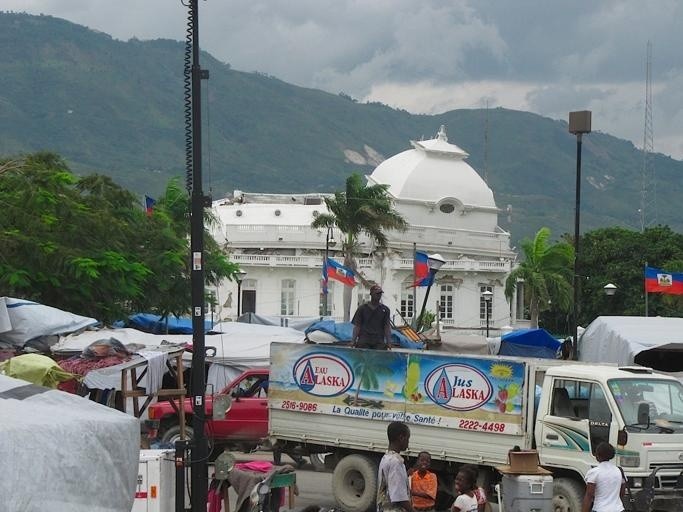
[145,368,268,467]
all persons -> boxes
[376,421,627,512]
[351,285,392,350]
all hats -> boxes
[370,285,384,294]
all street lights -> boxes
[324,222,336,316]
[232,268,248,318]
[604,282,619,315]
[415,251,447,335]
[483,290,493,338]
[569,110,593,362]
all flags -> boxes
[327,256,355,287]
[645,267,683,295]
[146,195,156,216]
[410,250,435,285]
[322,259,327,294]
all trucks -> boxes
[265,342,683,511]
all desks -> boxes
[222,471,296,512]
